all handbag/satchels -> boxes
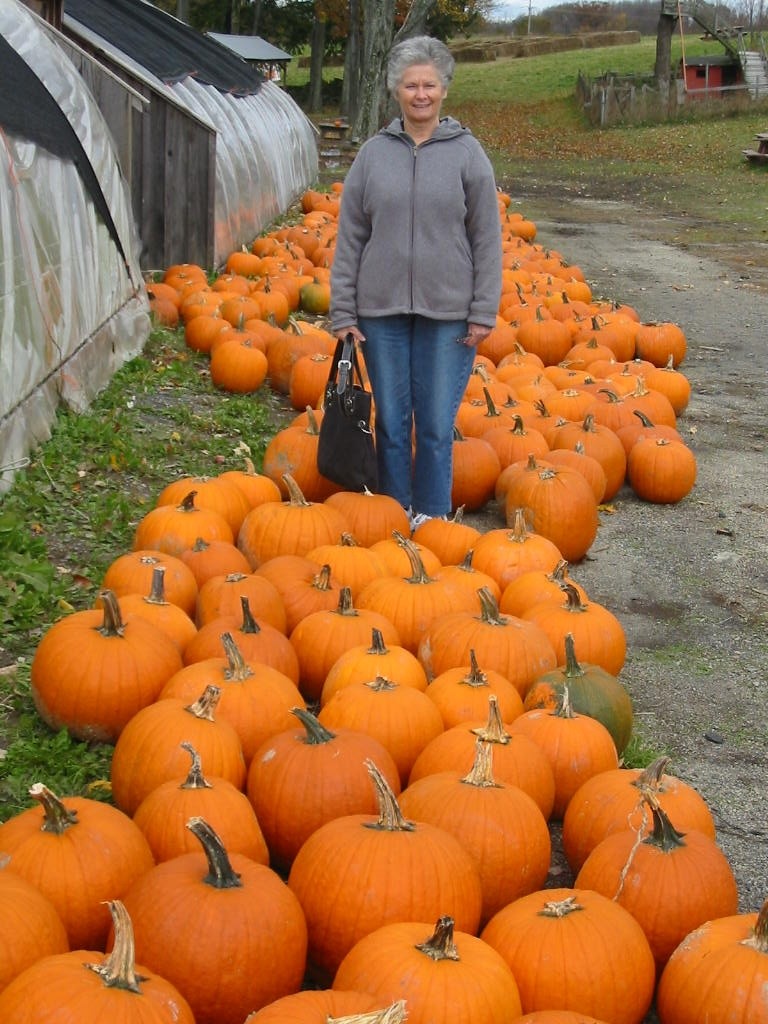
[317,381,378,490]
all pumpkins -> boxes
[143,182,375,501]
[413,187,696,564]
[1,456,768,1023]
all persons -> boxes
[329,35,503,535]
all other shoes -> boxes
[406,508,416,531]
[415,513,448,528]
